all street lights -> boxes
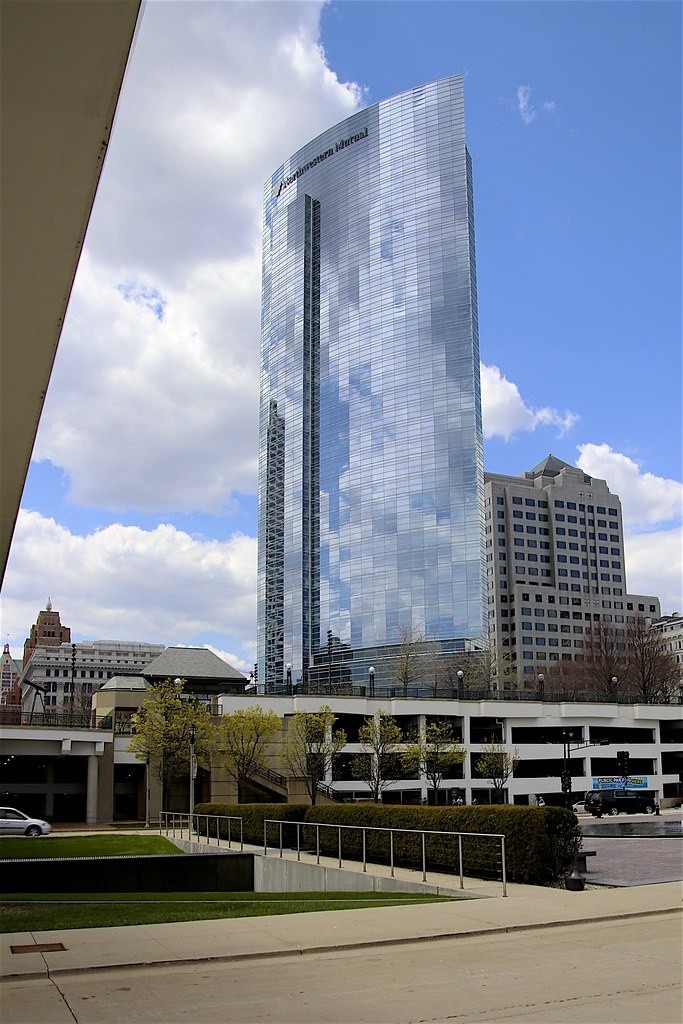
[253,663,258,695]
[457,670,464,699]
[612,676,618,702]
[327,629,333,695]
[69,644,77,725]
[369,666,376,698]
[538,673,545,694]
[285,663,292,695]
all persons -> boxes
[421,797,427,806]
[472,797,477,805]
[451,796,463,805]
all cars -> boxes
[0,807,53,835]
[572,801,588,813]
[584,790,656,816]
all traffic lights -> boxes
[618,751,630,776]
[561,769,573,792]
